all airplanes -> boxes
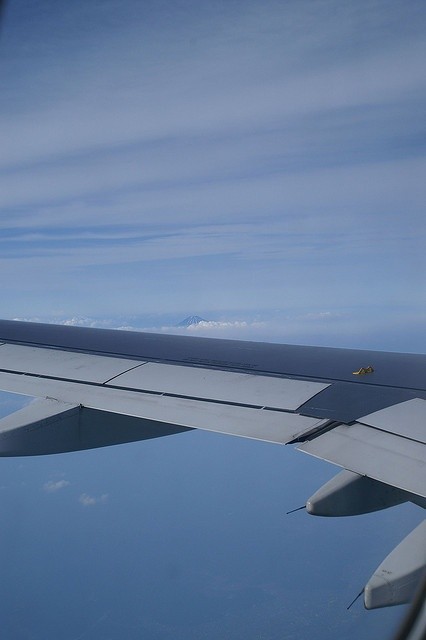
[0,319,426,640]
